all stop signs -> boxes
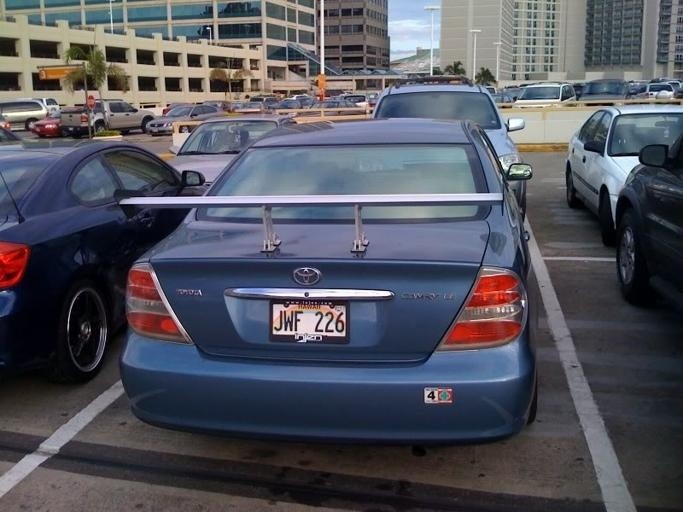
[87,94,96,110]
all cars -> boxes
[612,128,681,324]
[0,125,20,142]
[164,112,301,192]
[160,92,381,115]
[0,135,211,388]
[484,77,682,108]
[26,108,70,138]
[145,103,228,137]
[0,113,11,131]
[104,113,544,443]
[560,99,682,247]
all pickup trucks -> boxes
[60,98,156,141]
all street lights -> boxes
[206,26,211,45]
[491,42,504,80]
[469,29,482,80]
[423,5,440,75]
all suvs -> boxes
[369,78,530,228]
[29,96,60,117]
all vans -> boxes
[0,99,50,130]
